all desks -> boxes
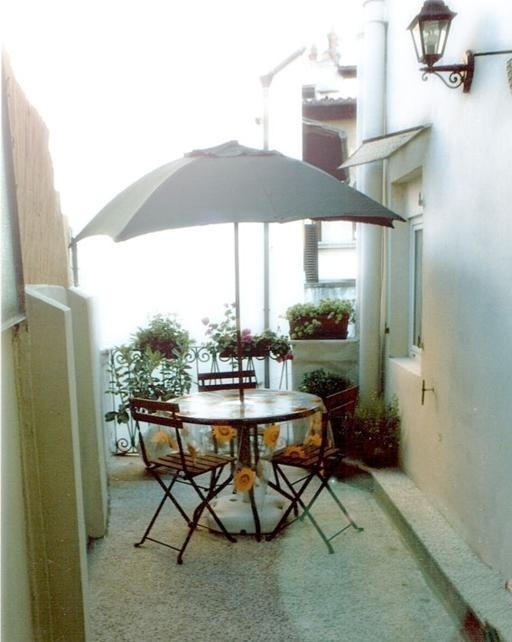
[166,387,322,538]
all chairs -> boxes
[196,370,273,476]
[128,398,237,564]
[266,384,360,555]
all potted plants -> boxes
[299,367,402,469]
[286,297,354,339]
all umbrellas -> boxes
[66,141,407,401]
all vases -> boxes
[220,338,269,357]
[139,334,179,359]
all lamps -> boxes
[405,0,475,93]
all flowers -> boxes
[200,300,296,363]
[127,311,192,359]
